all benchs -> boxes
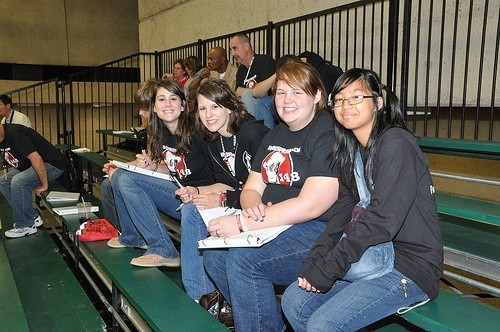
[0,129,500,332]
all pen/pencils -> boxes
[218,198,238,217]
[140,150,148,166]
[172,174,191,199]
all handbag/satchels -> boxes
[334,197,395,282]
[201,290,235,330]
[298,51,344,96]
[73,218,120,242]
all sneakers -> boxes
[13,215,43,228]
[131,253,181,267]
[108,237,148,249]
[5,224,38,237]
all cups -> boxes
[77,202,92,225]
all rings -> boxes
[215,230,221,236]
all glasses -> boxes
[331,95,378,108]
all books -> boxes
[108,159,175,181]
[46,191,80,200]
[71,148,89,153]
[198,223,291,248]
[53,206,99,216]
[112,130,134,134]
[175,189,242,227]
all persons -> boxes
[206,63,346,332]
[0,124,70,237]
[99,79,195,266]
[281,69,443,332]
[0,95,32,128]
[181,82,272,307]
[101,32,342,129]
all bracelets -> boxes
[195,186,199,194]
[219,191,227,207]
[235,215,246,231]
